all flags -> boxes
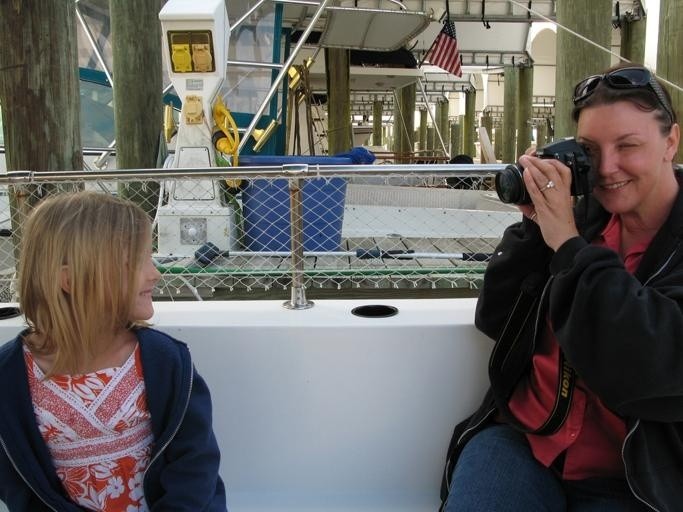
[423,15,463,79]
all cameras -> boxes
[494,138,599,204]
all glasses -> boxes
[572,67,674,124]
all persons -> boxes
[0,188,230,512]
[434,58,682,511]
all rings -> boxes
[538,180,556,192]
[530,212,536,221]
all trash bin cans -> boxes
[230,155,353,258]
